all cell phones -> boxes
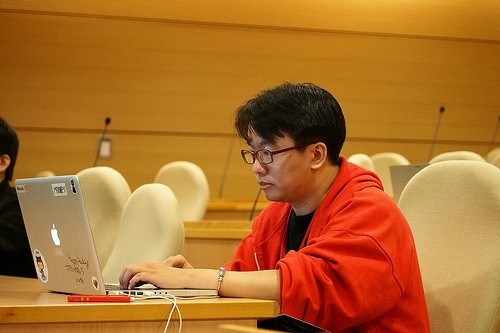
[68,295,131,302]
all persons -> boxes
[120,81,432,333]
[0,116,39,279]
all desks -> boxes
[0,275,280,333]
[205,200,271,220]
[180,219,251,270]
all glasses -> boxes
[240,146,297,165]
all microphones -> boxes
[94,116,111,166]
[427,106,446,161]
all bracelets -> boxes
[217,267,225,296]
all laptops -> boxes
[14,174,218,298]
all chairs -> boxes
[36,160,209,288]
[346,147,500,333]
